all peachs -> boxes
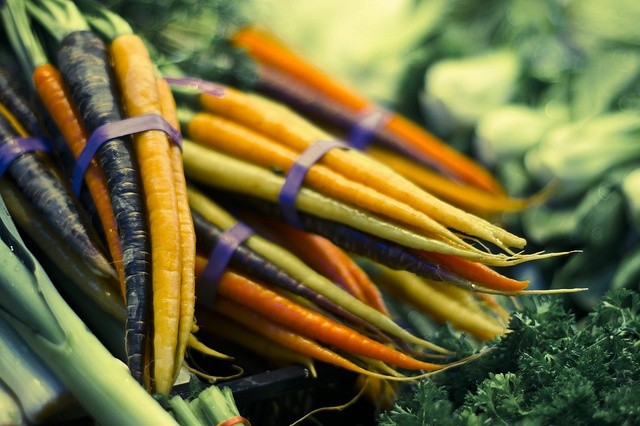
[1,240,181,426]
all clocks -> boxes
[419,0,639,167]
[505,168,640,285]
